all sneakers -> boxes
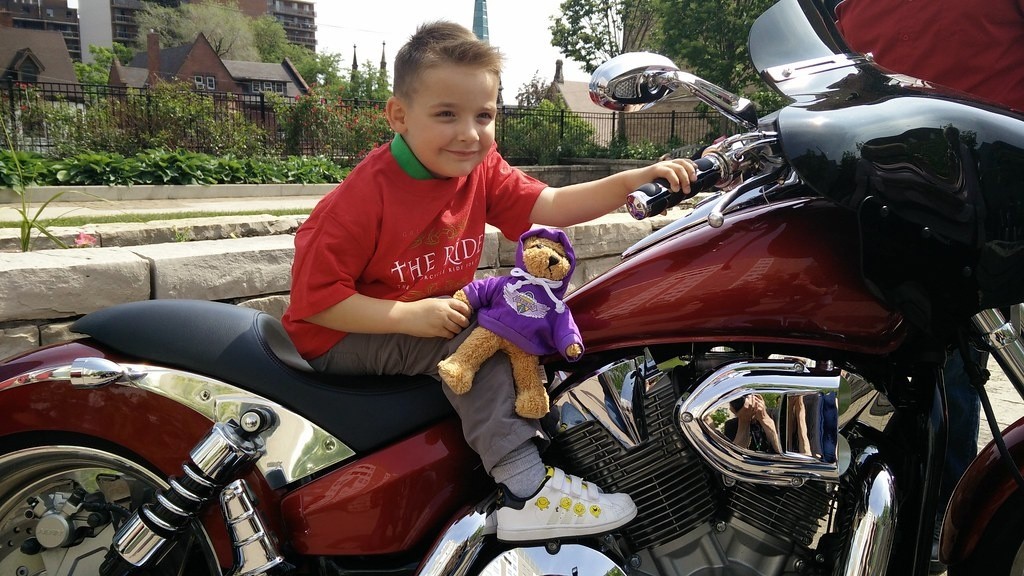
[496,465,638,543]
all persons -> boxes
[282,21,698,540]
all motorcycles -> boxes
[0,0,1023,576]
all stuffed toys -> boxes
[437,229,584,418]
